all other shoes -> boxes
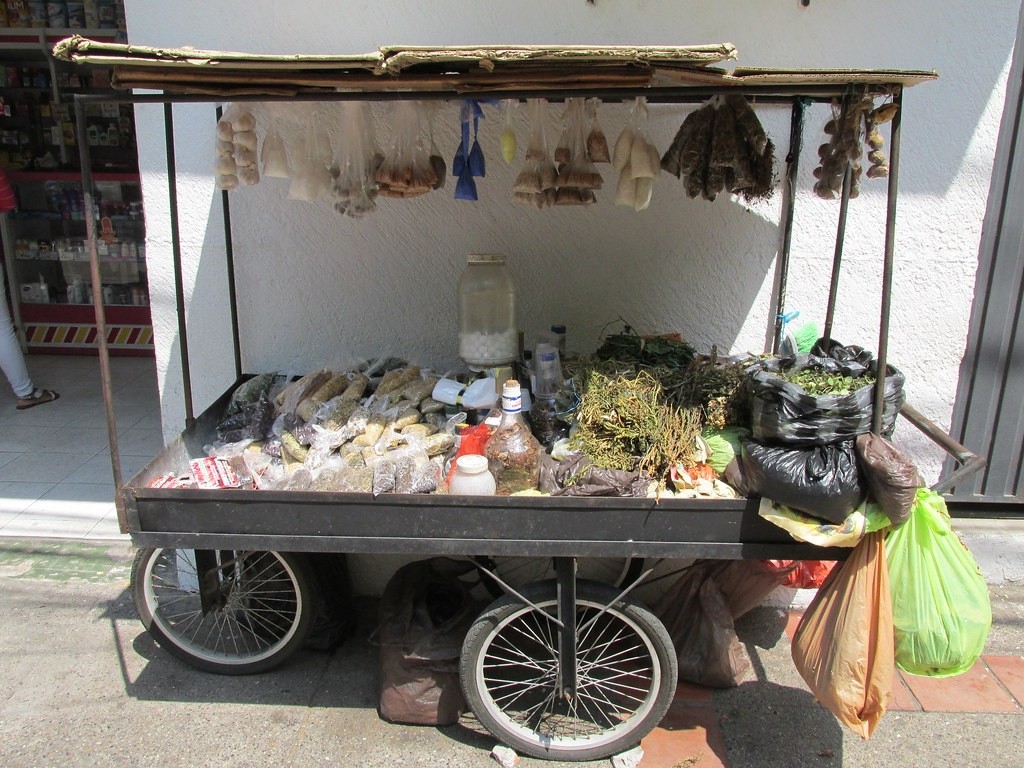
[16,388,60,409]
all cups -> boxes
[535,344,563,399]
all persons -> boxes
[0,171,59,409]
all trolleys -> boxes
[57,39,987,761]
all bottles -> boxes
[455,253,521,365]
[449,454,496,496]
[527,351,576,447]
[483,379,542,494]
[551,325,567,359]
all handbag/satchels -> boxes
[704,337,925,526]
[378,555,502,726]
[791,529,895,740]
[235,552,354,653]
[886,488,993,679]
[658,558,797,688]
